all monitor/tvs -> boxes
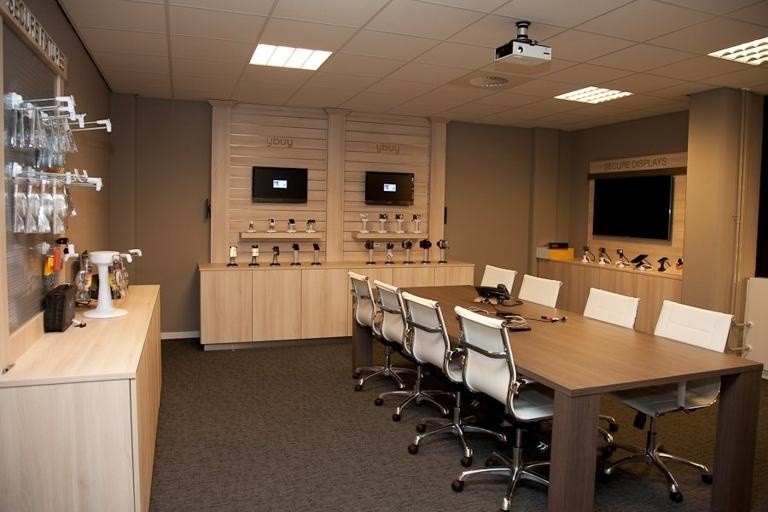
[593,176,673,239]
[252,165,307,204]
[364,171,416,206]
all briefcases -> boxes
[43,283,76,332]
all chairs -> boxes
[582,287,641,455]
[400,287,512,468]
[349,268,436,392]
[371,276,451,419]
[598,300,734,500]
[516,272,563,307]
[452,302,554,510]
[478,264,518,295]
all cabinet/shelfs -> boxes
[536,257,681,334]
[1,283,165,512]
[196,98,475,351]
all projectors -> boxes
[490,42,554,63]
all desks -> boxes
[350,284,764,512]
